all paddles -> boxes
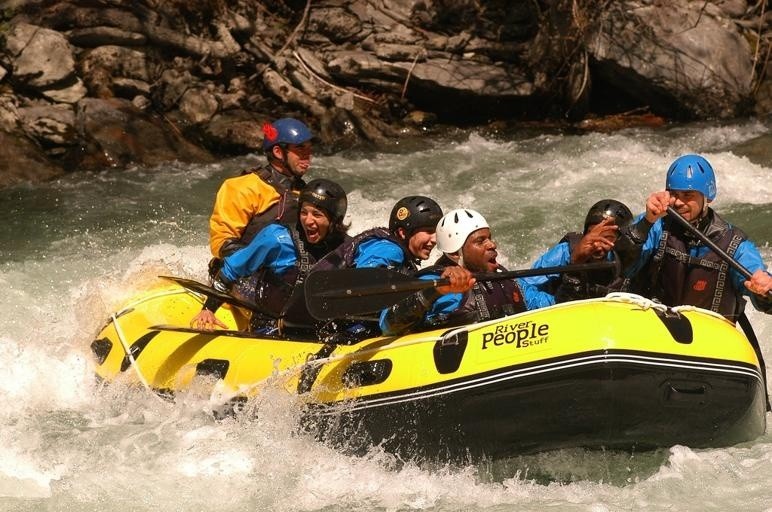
[148,324,285,350]
[304,261,619,321]
[157,271,281,322]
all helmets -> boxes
[436,209,490,253]
[390,196,442,233]
[666,156,716,201]
[263,118,316,150]
[297,179,347,234]
[583,200,633,237]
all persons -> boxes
[523,199,635,305]
[207,117,322,322]
[190,178,352,344]
[317,195,443,345]
[613,154,772,327]
[379,208,619,337]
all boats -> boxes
[89,273,769,477]
